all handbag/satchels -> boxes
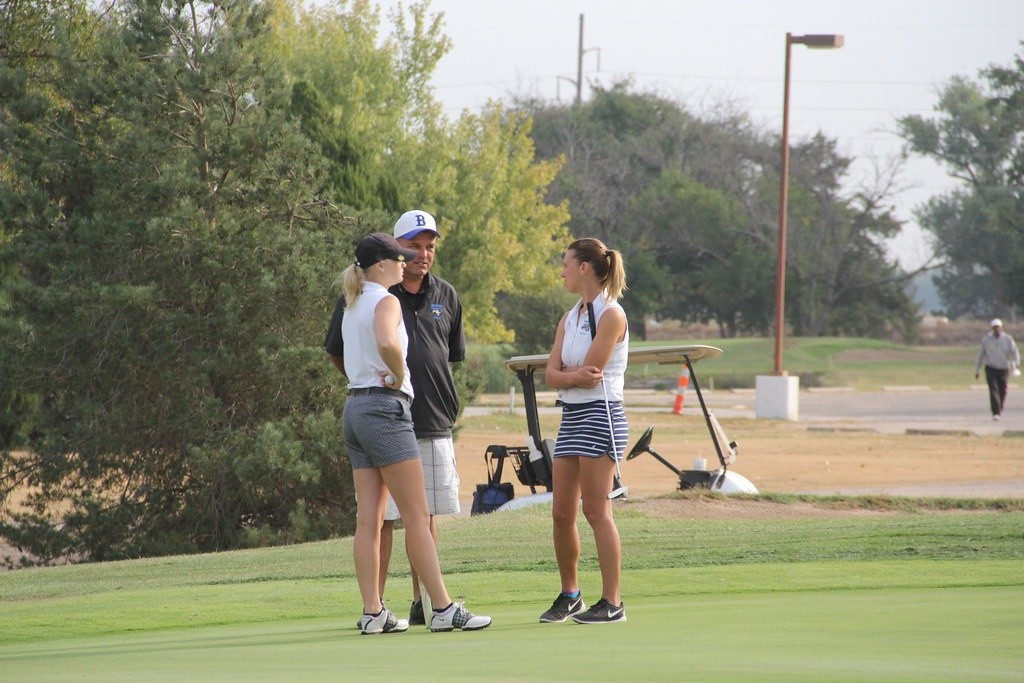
[470,445,514,516]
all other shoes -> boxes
[993,414,999,420]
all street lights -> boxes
[769,32,845,376]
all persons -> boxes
[974,318,1020,420]
[341,231,492,635]
[539,238,630,623]
[324,210,467,625]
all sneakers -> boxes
[430,602,492,632]
[357,599,384,628]
[361,606,409,635]
[539,590,586,623]
[408,596,426,625]
[571,598,627,624]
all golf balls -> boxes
[384,375,395,385]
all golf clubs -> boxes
[586,301,629,500]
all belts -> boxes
[349,386,411,405]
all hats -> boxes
[990,318,1002,327]
[393,210,440,240]
[354,233,418,269]
